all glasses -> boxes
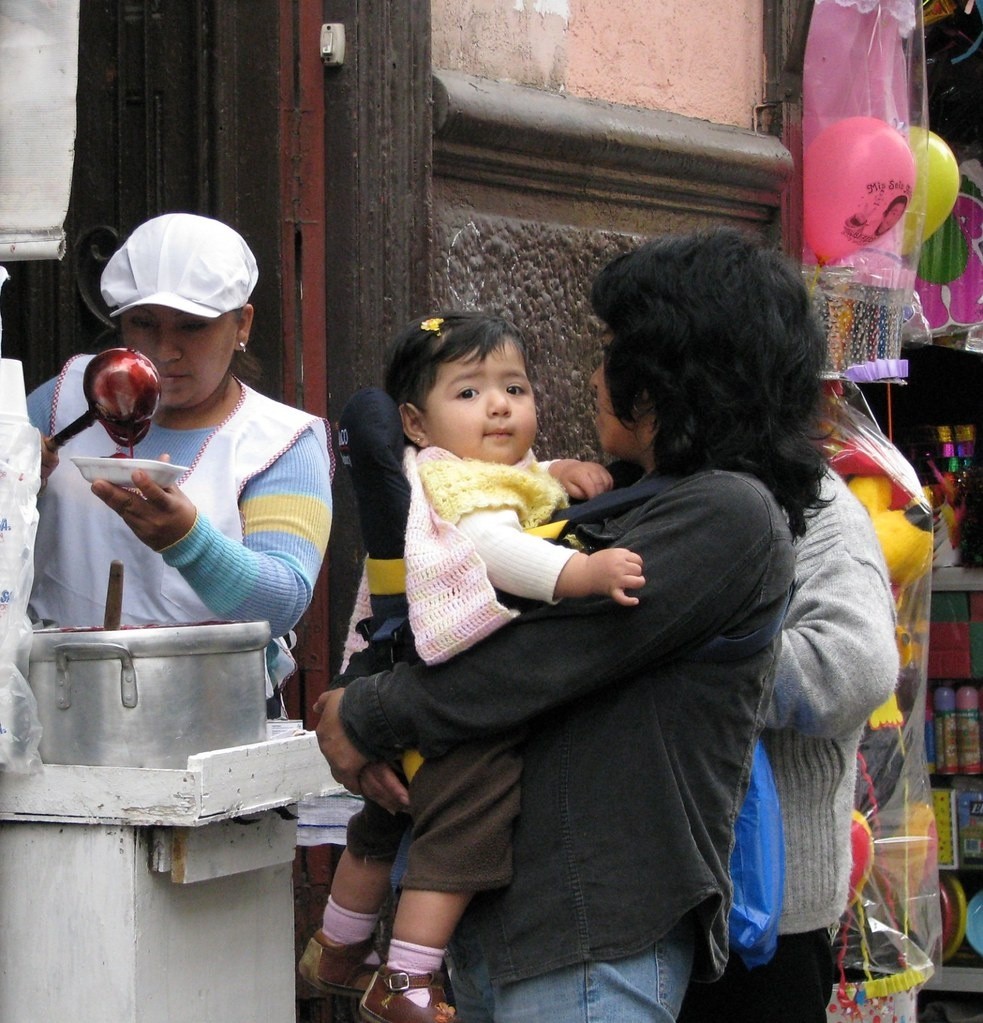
[589,339,609,375]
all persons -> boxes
[312,225,834,1023]
[677,462,898,1023]
[17,212,336,719]
[295,310,644,1023]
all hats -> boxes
[100,212,260,318]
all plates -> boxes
[69,456,193,489]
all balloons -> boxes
[800,117,916,265]
[903,124,961,260]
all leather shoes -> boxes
[297,934,385,999]
[359,962,462,1023]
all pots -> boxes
[29,617,273,770]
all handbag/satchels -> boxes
[729,738,785,970]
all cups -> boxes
[874,836,934,900]
